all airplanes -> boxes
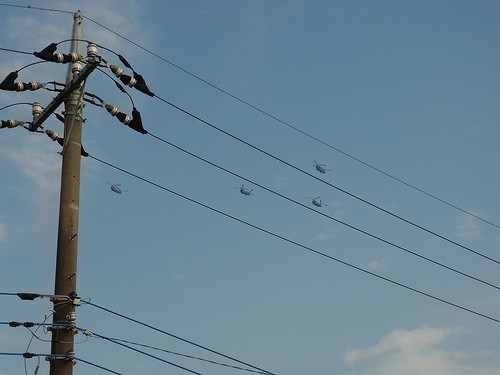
[233,183,255,197]
[306,195,328,208]
[312,160,336,174]
[104,181,130,194]
